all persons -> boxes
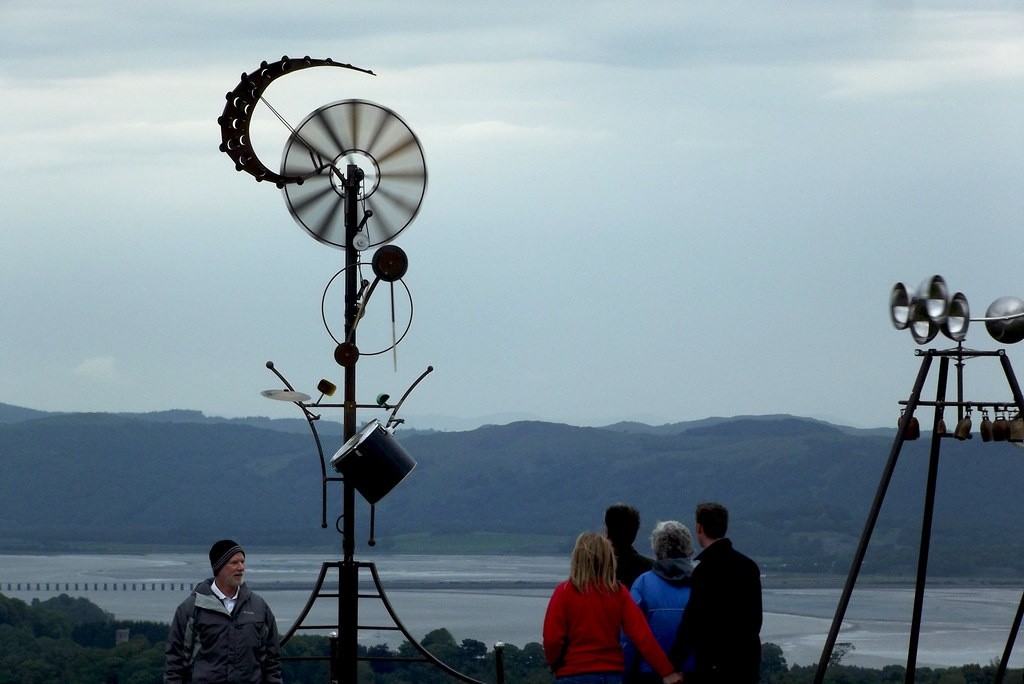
[163,539,283,684]
[543,502,763,684]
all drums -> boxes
[329,417,420,507]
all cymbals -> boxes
[260,387,312,403]
[888,273,1024,347]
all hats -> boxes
[209,540,246,577]
